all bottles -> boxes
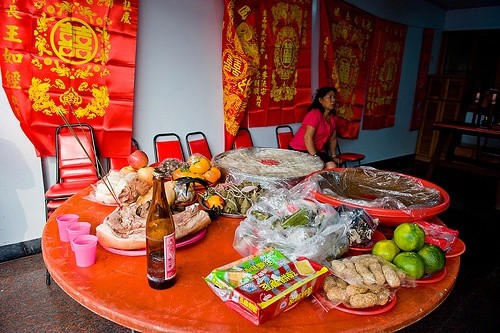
[146,170,176,290]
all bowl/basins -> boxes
[305,167,450,227]
[213,147,324,191]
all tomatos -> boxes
[172,158,222,187]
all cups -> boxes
[66,222,91,251]
[56,215,80,242]
[72,234,98,267]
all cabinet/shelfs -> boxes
[414,74,469,163]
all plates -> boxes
[150,162,175,182]
[316,284,398,315]
[444,237,466,257]
[404,257,447,284]
[199,191,248,218]
[99,225,208,257]
[192,176,220,189]
[348,229,386,251]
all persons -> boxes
[288,86,342,169]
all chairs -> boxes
[186,131,213,162]
[232,127,254,149]
[153,132,185,162]
[105,136,141,172]
[275,125,293,149]
[335,136,365,168]
[42,119,106,289]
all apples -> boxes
[119,150,156,185]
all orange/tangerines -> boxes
[371,222,446,280]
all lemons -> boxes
[208,195,224,209]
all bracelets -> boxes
[313,154,318,157]
[331,154,336,156]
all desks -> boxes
[41,164,462,333]
[423,120,500,209]
